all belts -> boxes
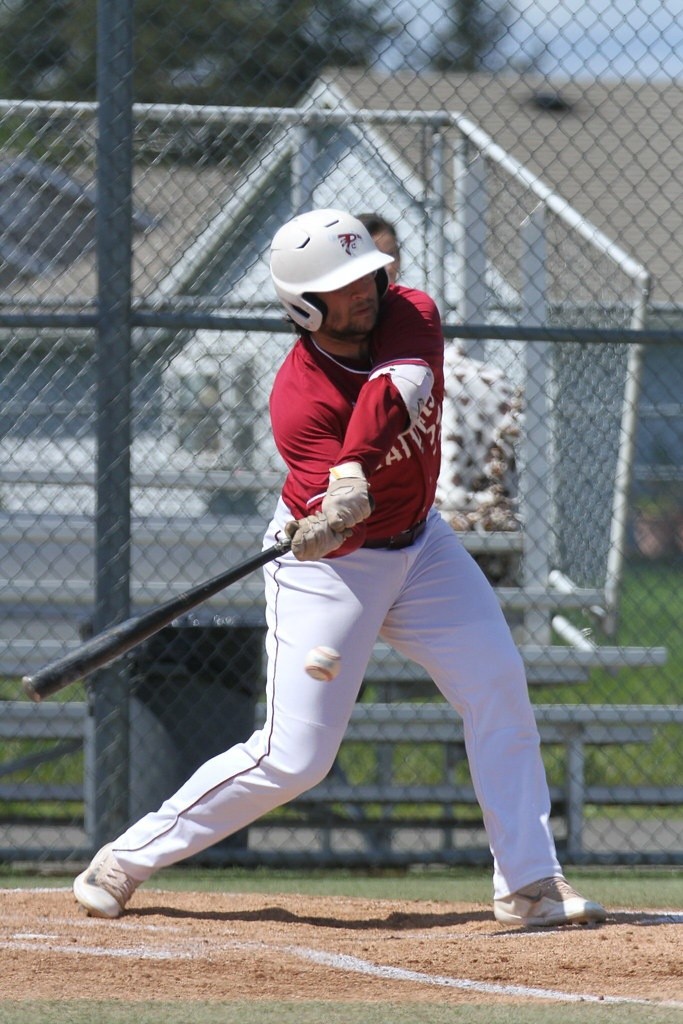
[361,513,426,551]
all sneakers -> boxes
[493,877,609,926]
[72,841,143,918]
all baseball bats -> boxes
[20,493,378,704]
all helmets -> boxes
[268,208,396,333]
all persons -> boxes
[354,212,401,286]
[71,206,605,925]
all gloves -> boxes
[282,509,346,562]
[321,477,372,533]
